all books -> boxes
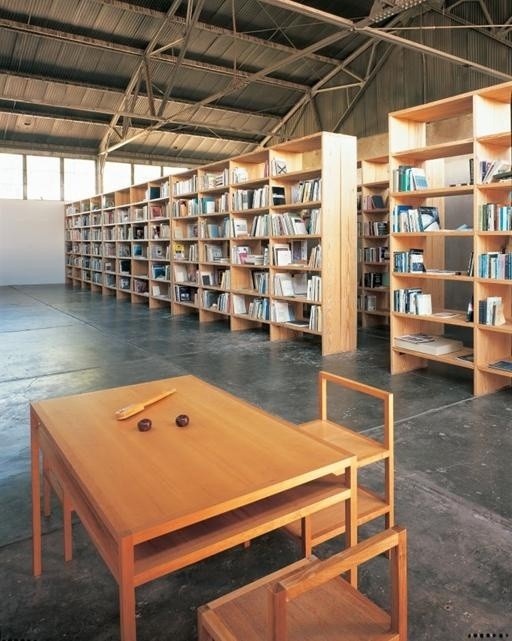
[65,196,116,287]
[199,169,230,312]
[270,157,321,331]
[118,208,131,290]
[132,189,149,293]
[356,185,391,313]
[150,181,171,298]
[230,159,269,320]
[392,154,512,375]
[173,175,199,304]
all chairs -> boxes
[29,370,409,641]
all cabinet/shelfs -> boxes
[63,79,512,398]
